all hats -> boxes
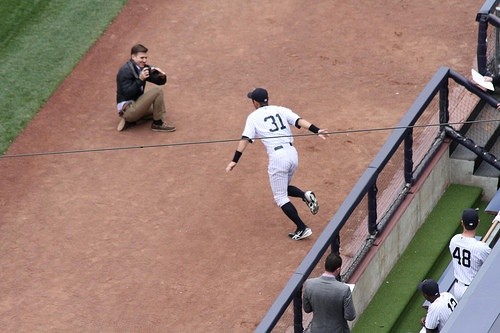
[463,209,478,230]
[418,279,439,295]
[247,88,269,103]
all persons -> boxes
[116,44,176,131]
[417,279,458,333]
[303,253,357,332]
[225,88,330,239]
[449,208,492,301]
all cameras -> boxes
[148,67,159,77]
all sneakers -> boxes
[150,122,176,132]
[288,227,312,241]
[117,118,127,131]
[303,191,319,215]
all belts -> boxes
[274,143,292,150]
[455,278,469,286]
